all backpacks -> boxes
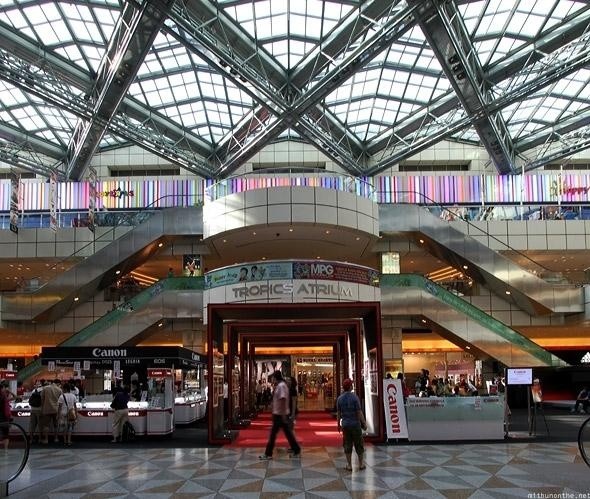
[122,421,135,444]
[28,388,43,408]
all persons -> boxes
[570,385,590,414]
[238,266,257,281]
[187,259,196,276]
[168,268,173,278]
[255,380,263,409]
[336,379,368,471]
[29,379,84,444]
[0,380,16,449]
[258,371,301,460]
[32,355,38,361]
[127,380,141,401]
[110,379,130,443]
[385,368,479,397]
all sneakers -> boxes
[256,453,303,462]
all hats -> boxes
[342,378,354,389]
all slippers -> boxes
[343,464,367,471]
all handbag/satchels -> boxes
[67,408,78,424]
